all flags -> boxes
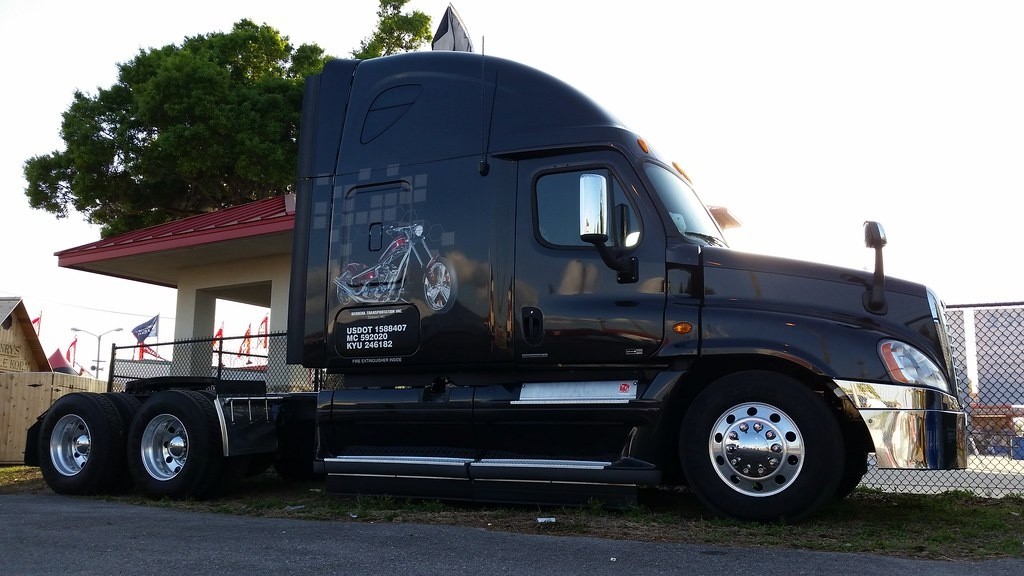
[238,323,251,358]
[31,315,41,332]
[213,321,225,348]
[66,333,78,363]
[257,316,268,349]
[132,313,159,343]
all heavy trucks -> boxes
[22,1,970,528]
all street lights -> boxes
[70,327,123,380]
